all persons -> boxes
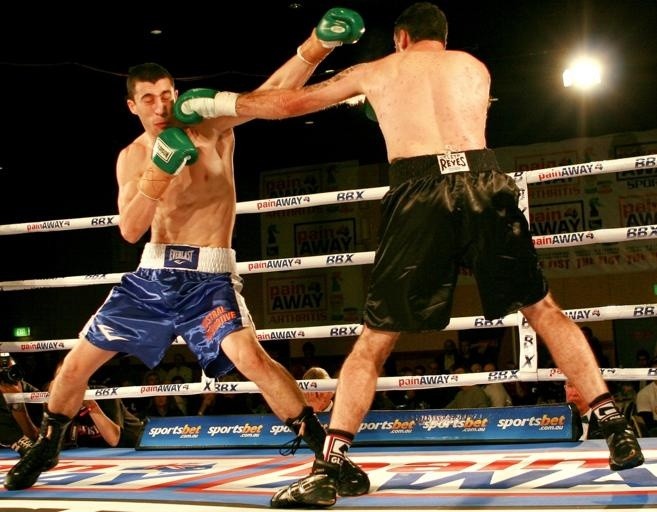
[172,1,643,509]
[130,370,257,416]
[303,368,334,412]
[637,381,657,438]
[44,358,143,448]
[0,354,44,450]
[5,7,370,497]
[381,338,603,440]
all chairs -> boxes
[624,401,643,438]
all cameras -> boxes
[0,352,25,383]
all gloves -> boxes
[150,127,198,177]
[173,88,239,124]
[296,6,366,67]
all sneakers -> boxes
[600,418,645,471]
[11,438,33,454]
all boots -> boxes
[285,405,369,498]
[4,405,73,490]
[271,451,370,507]
